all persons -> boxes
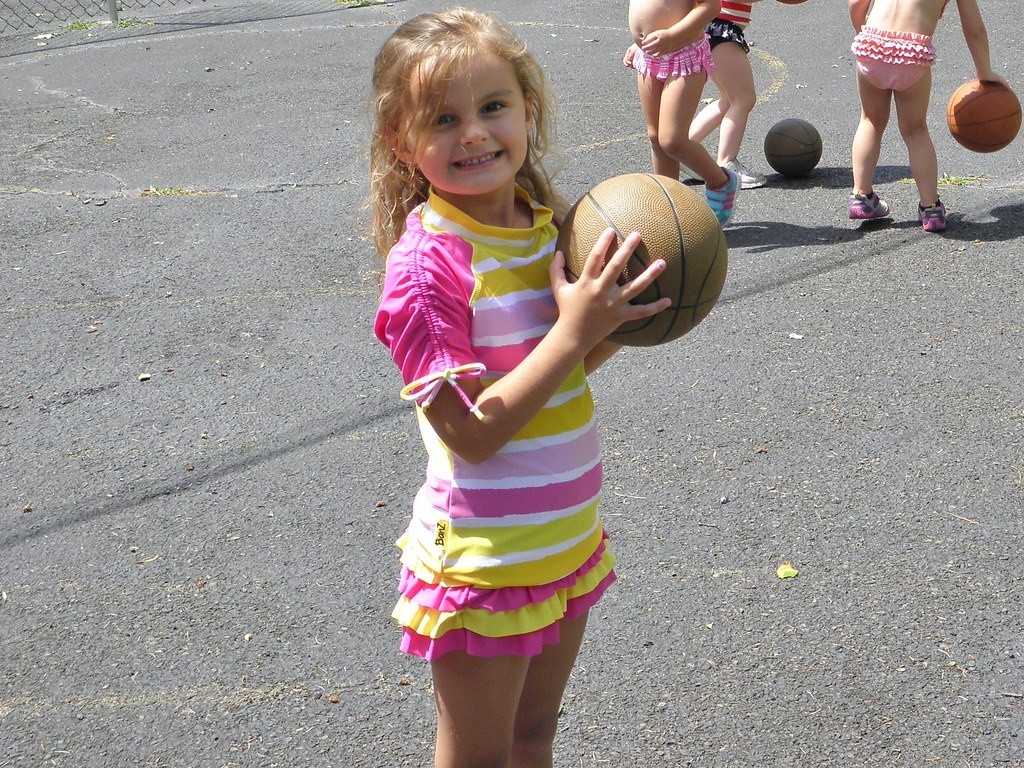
[622,2,742,234]
[369,7,674,767]
[847,0,1008,233]
[689,0,767,190]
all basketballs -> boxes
[946,79,1021,154]
[764,118,823,178]
[554,173,728,349]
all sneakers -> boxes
[849,188,891,219]
[704,167,742,229]
[918,199,946,231]
[680,162,704,180]
[717,159,767,189]
